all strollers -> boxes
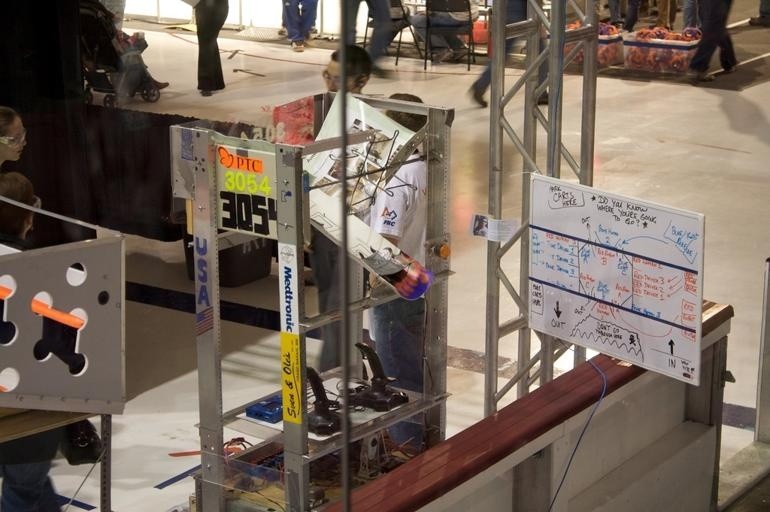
[81,0,169,108]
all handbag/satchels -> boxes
[59,419,103,466]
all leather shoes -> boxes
[470,82,489,108]
[537,90,550,106]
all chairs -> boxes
[361,0,477,71]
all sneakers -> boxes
[291,41,304,52]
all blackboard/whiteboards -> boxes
[527,172,705,387]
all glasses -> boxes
[323,71,354,83]
[30,194,41,209]
[0,128,27,148]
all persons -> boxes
[0,106,27,173]
[608,0,770,82]
[344,0,399,79]
[308,43,372,372]
[408,0,480,64]
[471,0,548,107]
[370,93,428,464]
[282,0,318,51]
[0,173,62,512]
[194,0,228,96]
